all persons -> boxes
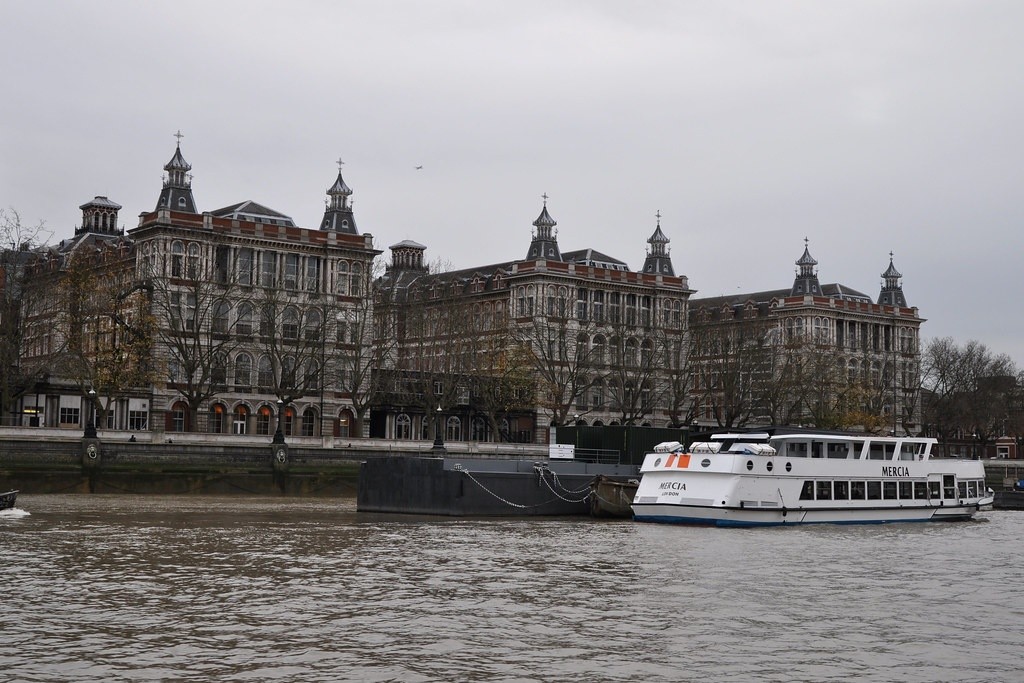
[166,436,173,443]
[127,435,138,444]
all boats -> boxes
[630,432,995,525]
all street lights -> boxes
[272,395,285,444]
[972,433,976,459]
[693,420,698,442]
[431,404,448,450]
[82,389,98,438]
[573,413,579,447]
